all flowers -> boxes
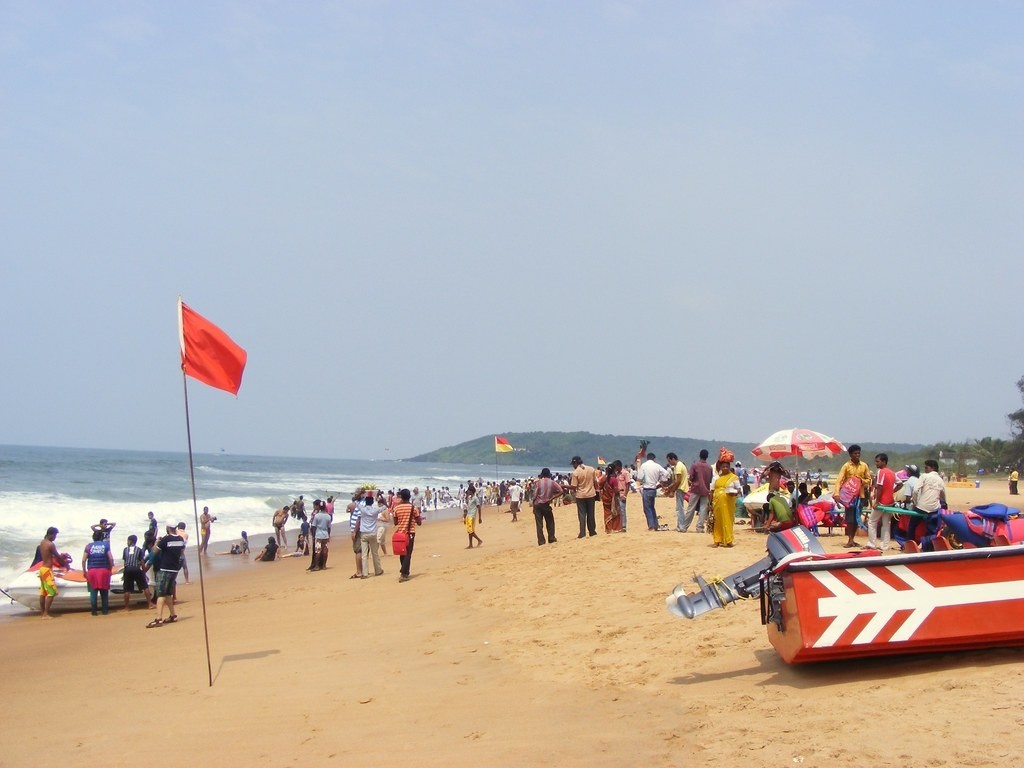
[360,483,378,490]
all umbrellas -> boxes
[752,428,847,512]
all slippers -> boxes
[844,541,860,548]
[350,574,358,579]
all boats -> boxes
[0,557,157,611]
[665,525,1024,670]
[741,484,793,522]
[876,503,1024,546]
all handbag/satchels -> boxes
[392,533,410,556]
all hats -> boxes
[166,520,178,529]
[905,464,920,474]
[569,456,581,466]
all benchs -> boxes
[817,510,872,534]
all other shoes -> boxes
[657,524,669,531]
[399,574,408,582]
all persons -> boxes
[40,527,71,619]
[279,534,309,558]
[89,519,116,545]
[255,537,278,561]
[300,516,309,540]
[199,506,217,553]
[306,495,339,571]
[290,496,306,519]
[561,447,749,548]
[731,461,844,537]
[832,443,947,548]
[948,473,966,482]
[272,506,289,549]
[142,511,191,604]
[83,530,114,615]
[346,467,573,580]
[146,524,184,628]
[1008,468,1019,495]
[216,531,250,555]
[123,535,157,610]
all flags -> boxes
[597,457,606,465]
[495,437,513,452]
[178,296,247,395]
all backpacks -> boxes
[832,475,862,509]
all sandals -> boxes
[146,618,163,628]
[164,616,178,623]
